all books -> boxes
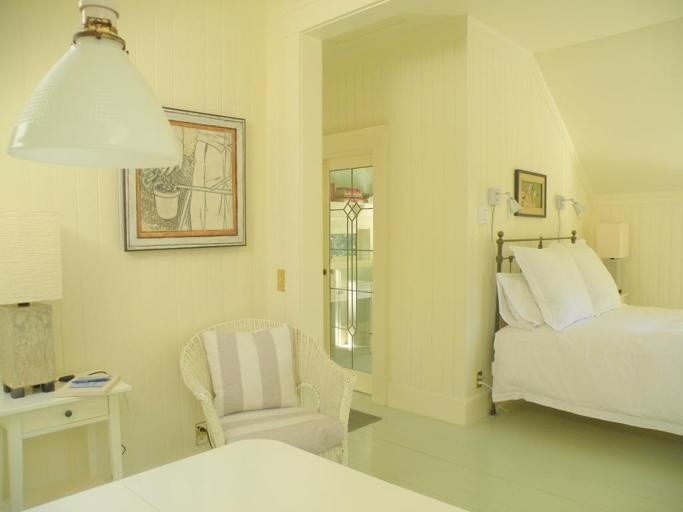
[54,374,122,398]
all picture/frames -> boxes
[119,104,247,252]
[514,168,548,219]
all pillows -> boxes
[203,323,300,418]
[495,236,621,332]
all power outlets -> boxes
[477,372,483,389]
[195,422,209,445]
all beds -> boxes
[489,231,683,442]
[21,438,488,512]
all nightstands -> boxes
[0,373,131,511]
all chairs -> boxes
[178,317,355,467]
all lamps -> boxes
[8,1,184,169]
[0,214,64,399]
[555,194,585,218]
[487,187,522,219]
